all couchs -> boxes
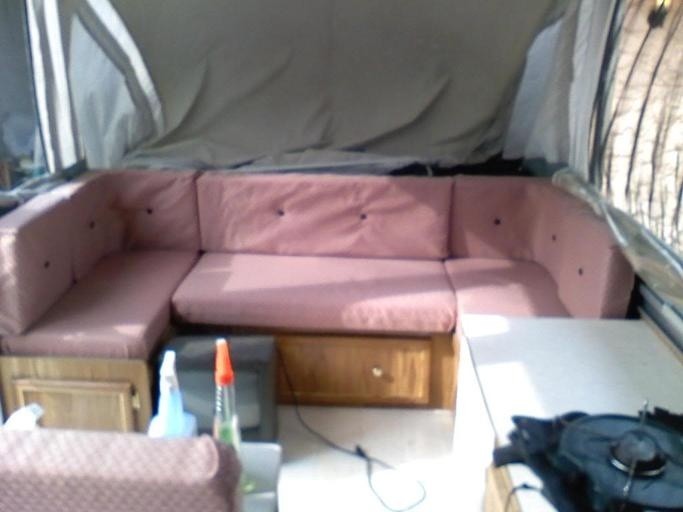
[428,170,637,413]
[0,159,203,431]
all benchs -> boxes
[167,164,460,414]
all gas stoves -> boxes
[494,397,683,512]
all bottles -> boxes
[211,338,258,494]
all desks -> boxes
[231,437,286,511]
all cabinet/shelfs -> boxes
[439,298,682,512]
